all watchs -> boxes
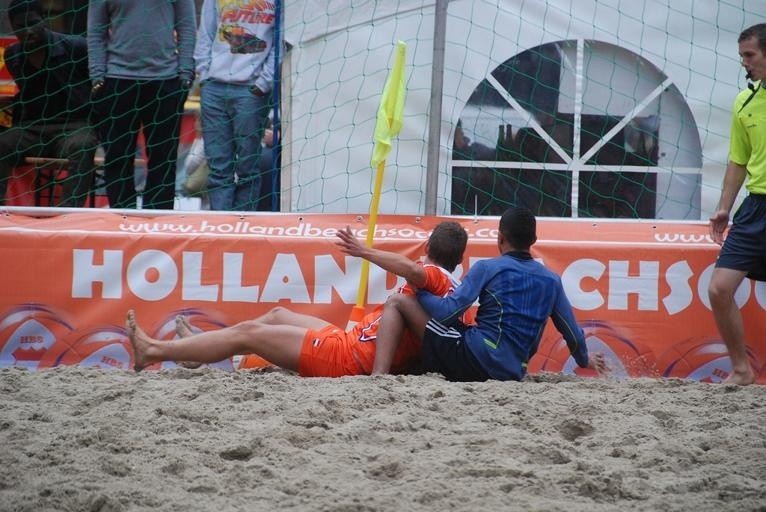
[250,84,263,96]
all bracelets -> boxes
[91,82,103,93]
[182,80,194,88]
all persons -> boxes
[126,220,467,379]
[710,23,766,386]
[98,111,280,211]
[192,0,283,211]
[369,206,607,379]
[0,0,97,207]
[89,0,197,209]
